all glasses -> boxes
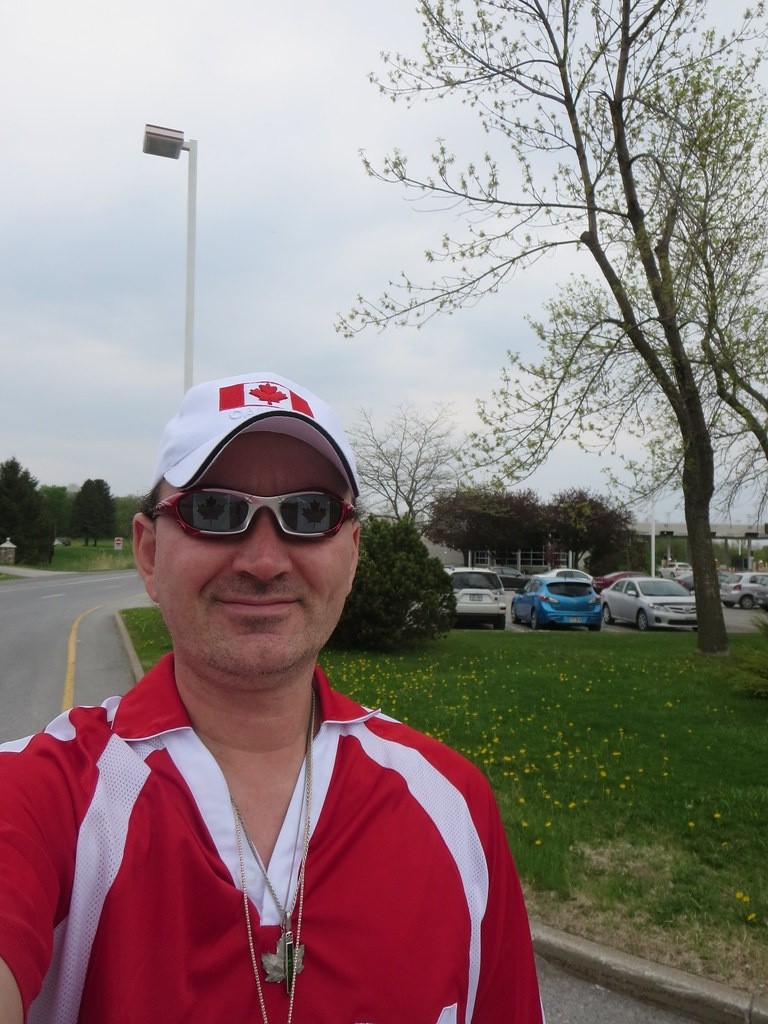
[151,488,358,536]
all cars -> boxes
[590,571,650,591]
[535,570,593,582]
[485,565,530,591]
[510,573,603,631]
[601,577,698,630]
[658,562,692,578]
[719,572,768,608]
[54,539,62,545]
[676,571,731,591]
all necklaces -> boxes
[232,689,316,1024]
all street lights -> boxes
[647,440,663,577]
[142,126,197,395]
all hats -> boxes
[148,371,361,507]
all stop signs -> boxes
[113,537,123,546]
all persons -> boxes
[0,372,546,1024]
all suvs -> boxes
[440,565,506,631]
